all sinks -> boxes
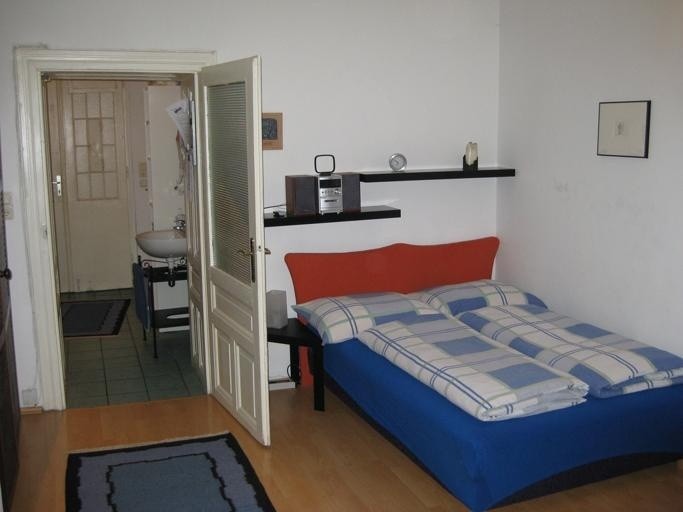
[135,230,187,258]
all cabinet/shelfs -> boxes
[144,86,185,231]
[264,166,515,227]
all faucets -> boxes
[173,219,185,231]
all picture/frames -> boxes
[597,100,651,158]
[261,113,283,150]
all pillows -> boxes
[291,279,548,346]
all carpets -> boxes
[65,430,277,512]
[60,298,131,337]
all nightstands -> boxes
[268,318,325,412]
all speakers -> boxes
[285,172,361,217]
[267,290,288,330]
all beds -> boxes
[285,236,683,512]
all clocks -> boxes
[389,153,407,171]
